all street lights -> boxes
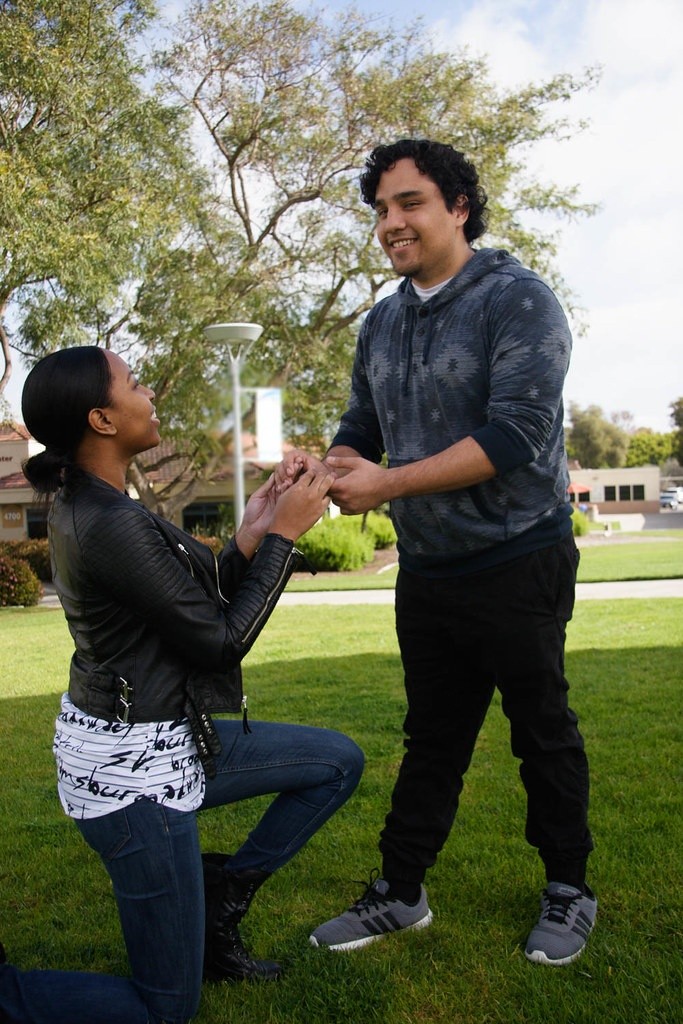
[203,321,266,534]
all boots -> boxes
[200,852,283,982]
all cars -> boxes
[660,486,683,509]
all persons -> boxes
[0,344,367,1024]
[272,136,602,966]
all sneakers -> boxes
[308,877,434,951]
[525,880,599,966]
